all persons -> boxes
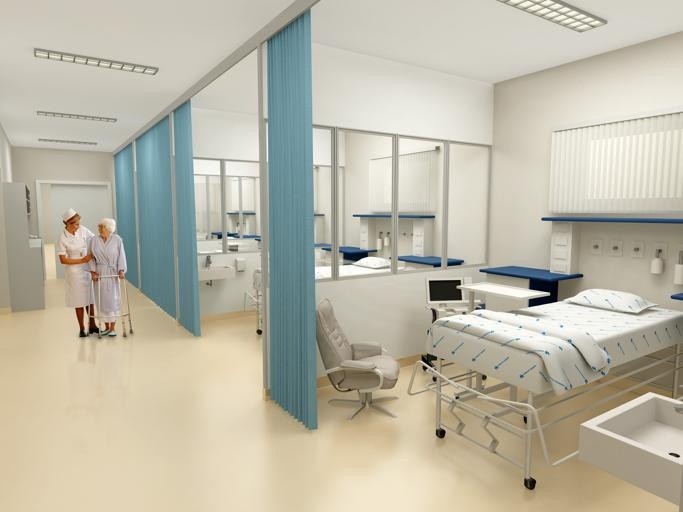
[56,207,101,337]
[89,218,128,337]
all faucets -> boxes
[205,256,212,268]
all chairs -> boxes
[316,298,400,419]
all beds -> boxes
[252,265,405,337]
[421,305,681,490]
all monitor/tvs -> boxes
[427,278,464,303]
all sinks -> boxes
[197,265,236,282]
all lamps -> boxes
[34,47,158,76]
[498,0,608,35]
[650,247,665,274]
[674,250,682,283]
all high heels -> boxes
[89,328,99,333]
[80,329,89,337]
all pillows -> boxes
[566,288,657,314]
[353,257,391,269]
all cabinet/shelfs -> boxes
[2,181,46,313]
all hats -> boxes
[62,208,76,222]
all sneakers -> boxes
[101,329,109,335]
[108,330,116,336]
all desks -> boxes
[208,230,464,272]
[477,264,583,315]
[450,281,551,400]
